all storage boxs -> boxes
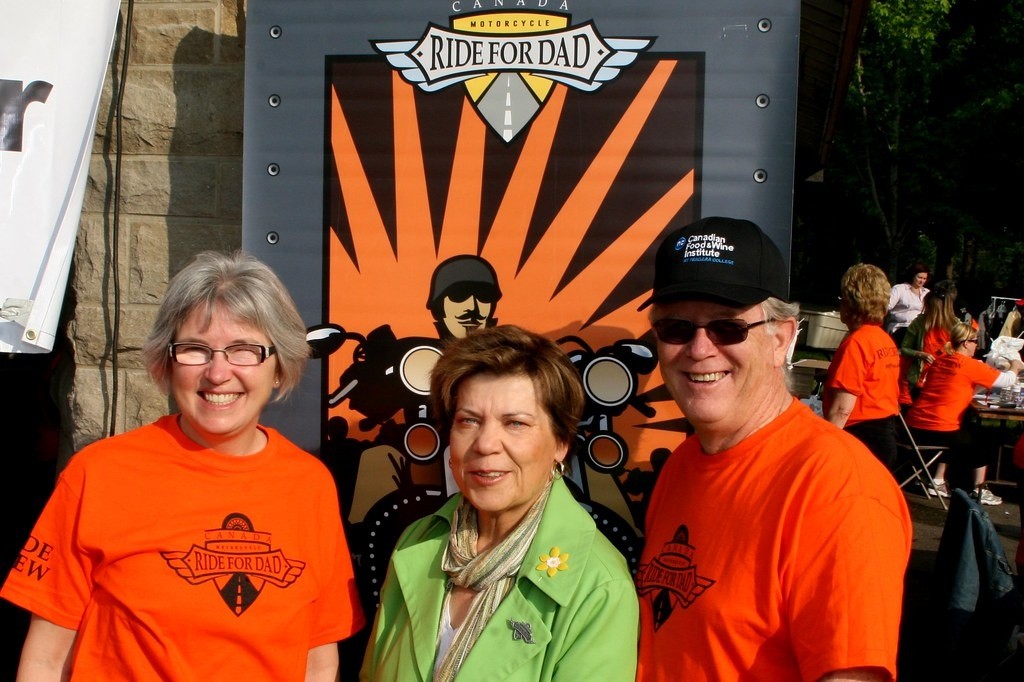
[797,310,849,349]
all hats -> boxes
[637,216,788,313]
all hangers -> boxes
[991,297,1018,310]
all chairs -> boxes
[896,411,950,511]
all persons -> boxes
[823,263,1024,507]
[358,326,641,682]
[0,250,367,682]
[637,217,914,682]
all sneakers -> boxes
[928,480,952,499]
[974,484,1002,506]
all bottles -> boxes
[1000,377,1024,409]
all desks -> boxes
[792,359,833,399]
[970,399,1024,486]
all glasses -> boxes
[961,338,978,344]
[167,340,276,367]
[651,317,776,345]
[930,289,945,302]
[837,296,844,303]
